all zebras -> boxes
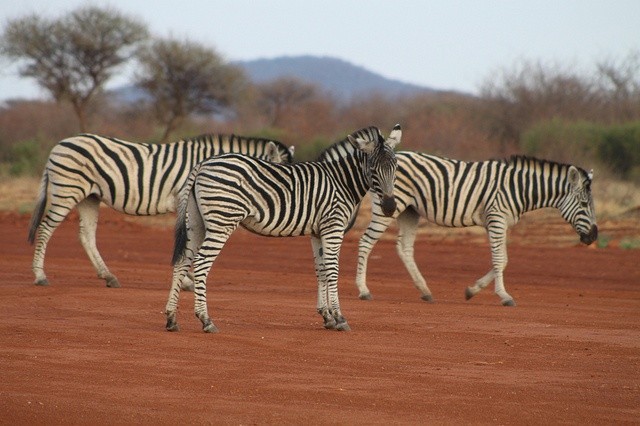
[165,124,402,332]
[355,151,598,306]
[27,132,295,289]
